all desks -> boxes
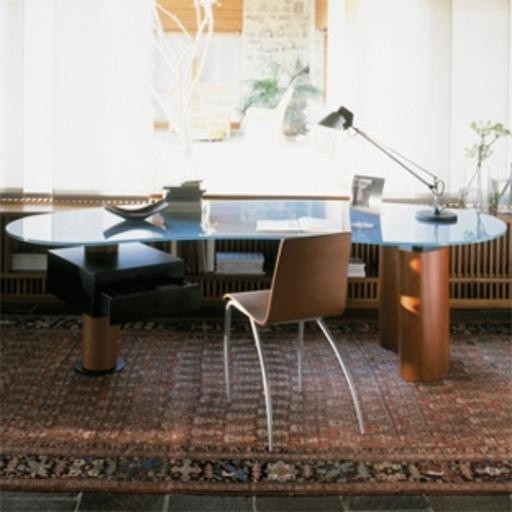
[4,199,510,383]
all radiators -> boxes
[0,194,509,308]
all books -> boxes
[350,173,389,209]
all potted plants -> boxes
[456,118,510,209]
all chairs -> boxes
[222,231,366,450]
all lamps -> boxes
[317,105,459,224]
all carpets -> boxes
[0,311,511,495]
